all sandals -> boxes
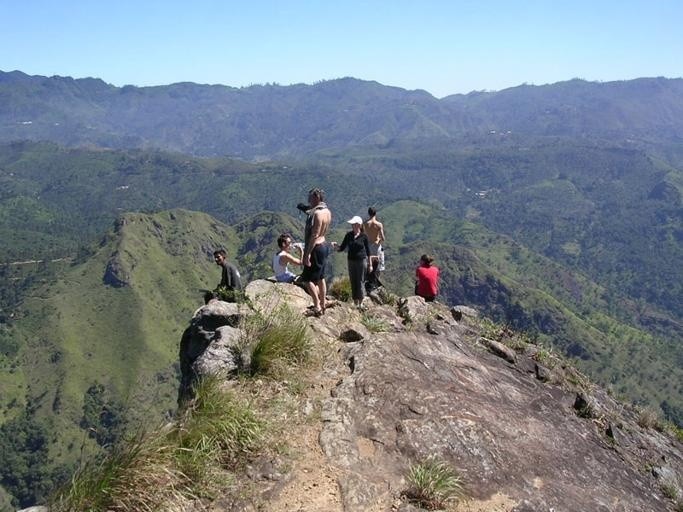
[306,305,325,314]
[304,308,323,317]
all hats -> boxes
[346,216,362,224]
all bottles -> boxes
[290,242,305,250]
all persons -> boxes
[361,208,384,256]
[297,187,331,319]
[375,242,389,283]
[414,252,442,302]
[272,234,303,283]
[202,247,244,300]
[338,217,373,304]
